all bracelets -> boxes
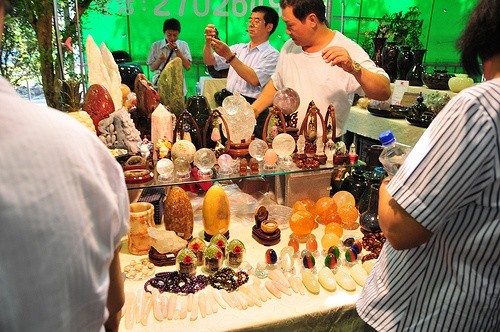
[172,47,179,53]
[225,53,237,64]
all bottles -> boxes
[371,37,388,69]
[426,70,455,89]
[360,183,381,233]
[407,50,426,86]
[412,92,427,119]
[397,46,414,80]
[448,74,475,93]
[378,130,409,179]
[382,42,398,83]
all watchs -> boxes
[349,60,362,75]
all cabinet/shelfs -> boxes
[102,156,366,332]
[346,83,458,173]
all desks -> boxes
[198,76,226,109]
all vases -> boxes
[372,37,429,86]
[342,169,383,232]
[184,95,213,147]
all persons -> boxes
[251,0,392,144]
[203,5,281,140]
[350,0,500,332]
[0,0,132,332]
[146,18,192,104]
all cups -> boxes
[127,202,155,255]
